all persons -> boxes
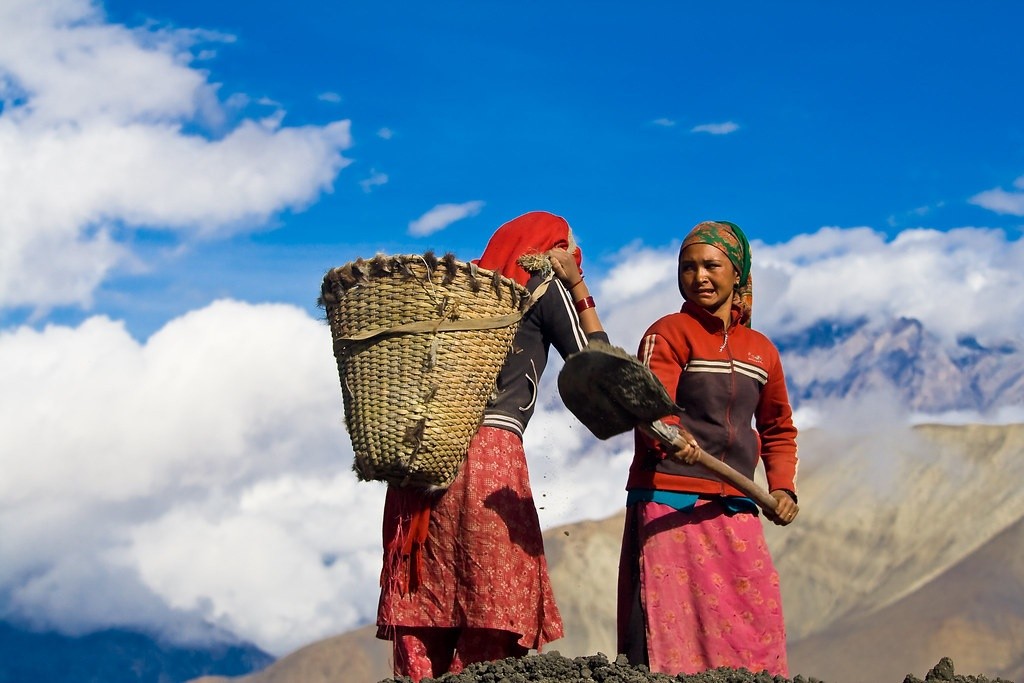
[617,221,799,678]
[376,211,609,683]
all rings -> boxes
[788,512,792,517]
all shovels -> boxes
[557,349,779,513]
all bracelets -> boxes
[576,295,595,314]
[566,278,583,291]
